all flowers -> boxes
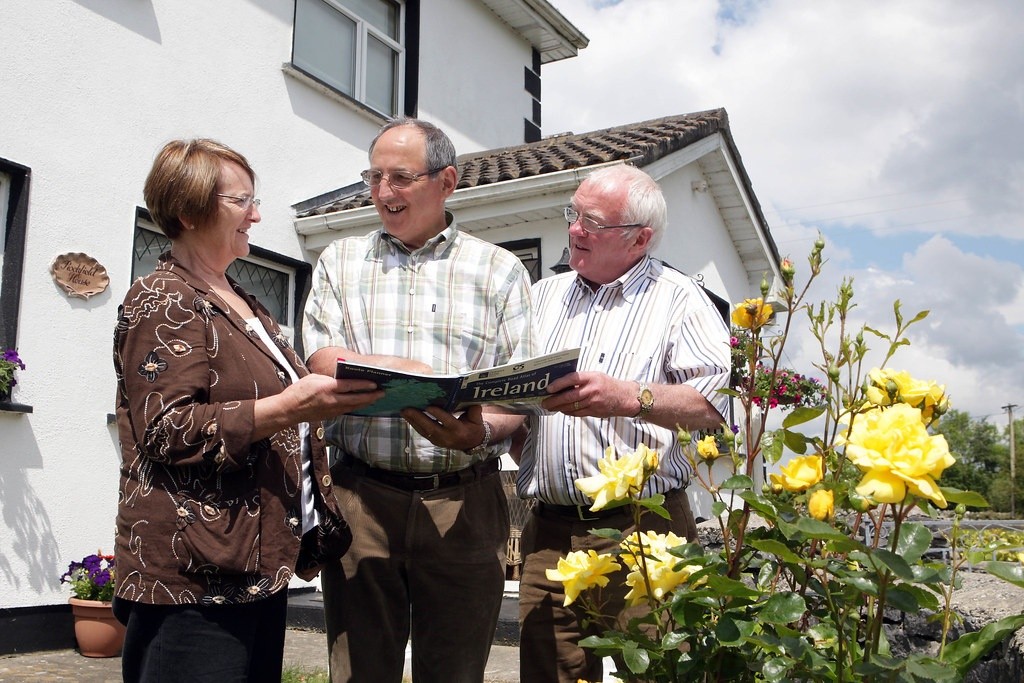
[546,228,1024,683]
[0,343,25,394]
[60,550,118,601]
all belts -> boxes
[538,487,680,520]
[336,446,499,492]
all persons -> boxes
[301,118,533,683]
[114,139,387,683]
[509,165,733,683]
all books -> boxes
[334,347,582,418]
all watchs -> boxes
[466,422,491,455]
[633,381,654,419]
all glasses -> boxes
[217,193,261,210]
[564,206,645,233]
[360,165,447,189]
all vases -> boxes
[0,378,17,402]
[69,593,123,659]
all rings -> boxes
[427,434,431,438]
[574,402,579,410]
